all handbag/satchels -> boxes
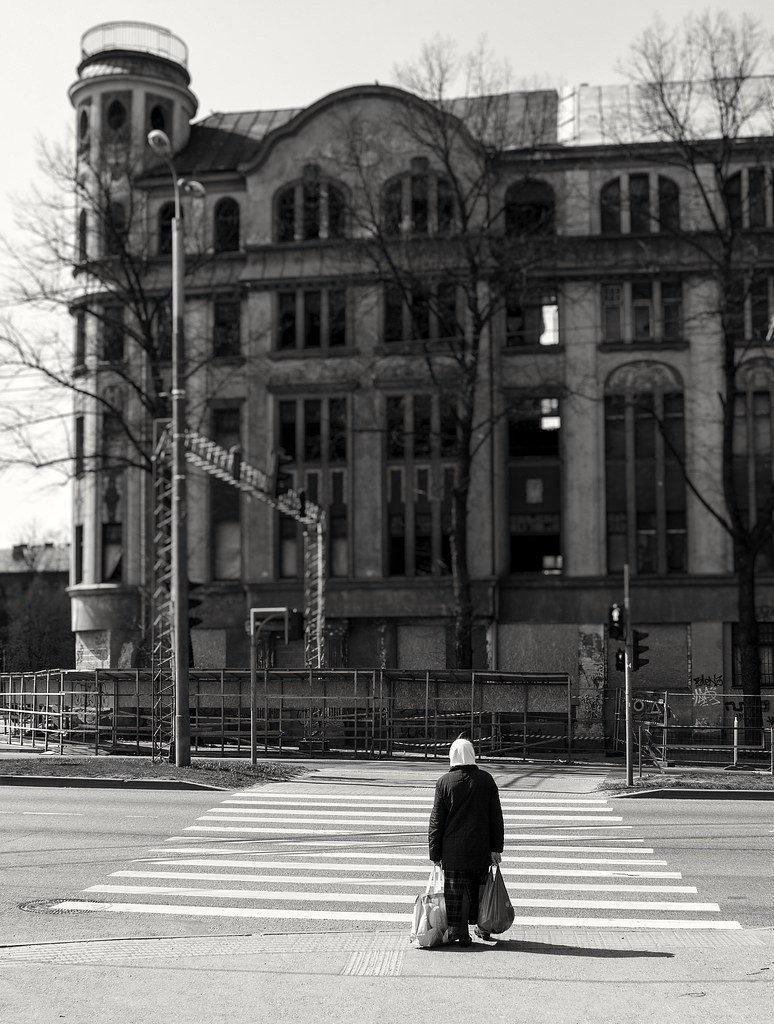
[411,860,447,948]
[477,859,515,934]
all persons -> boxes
[428,739,504,948]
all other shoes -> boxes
[473,925,490,940]
[459,932,472,948]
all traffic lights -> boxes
[616,650,625,672]
[608,603,624,639]
[633,628,649,672]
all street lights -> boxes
[145,126,205,777]
